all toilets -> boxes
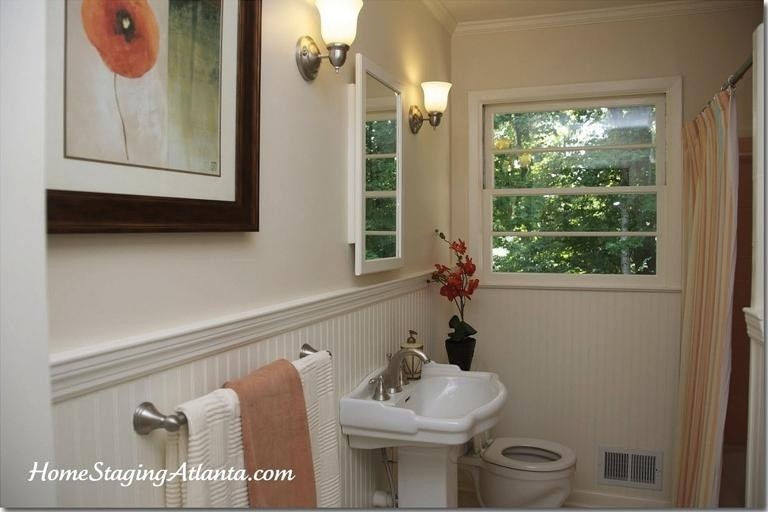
[457,431,577,509]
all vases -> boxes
[444,315,478,370]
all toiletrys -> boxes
[399,329,423,381]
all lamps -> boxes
[409,81,453,135]
[295,0,364,82]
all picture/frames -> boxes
[46,0,261,234]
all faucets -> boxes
[381,348,430,394]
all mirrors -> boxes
[347,52,406,276]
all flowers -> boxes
[430,229,479,321]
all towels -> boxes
[164,350,342,512]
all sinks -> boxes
[339,358,507,452]
